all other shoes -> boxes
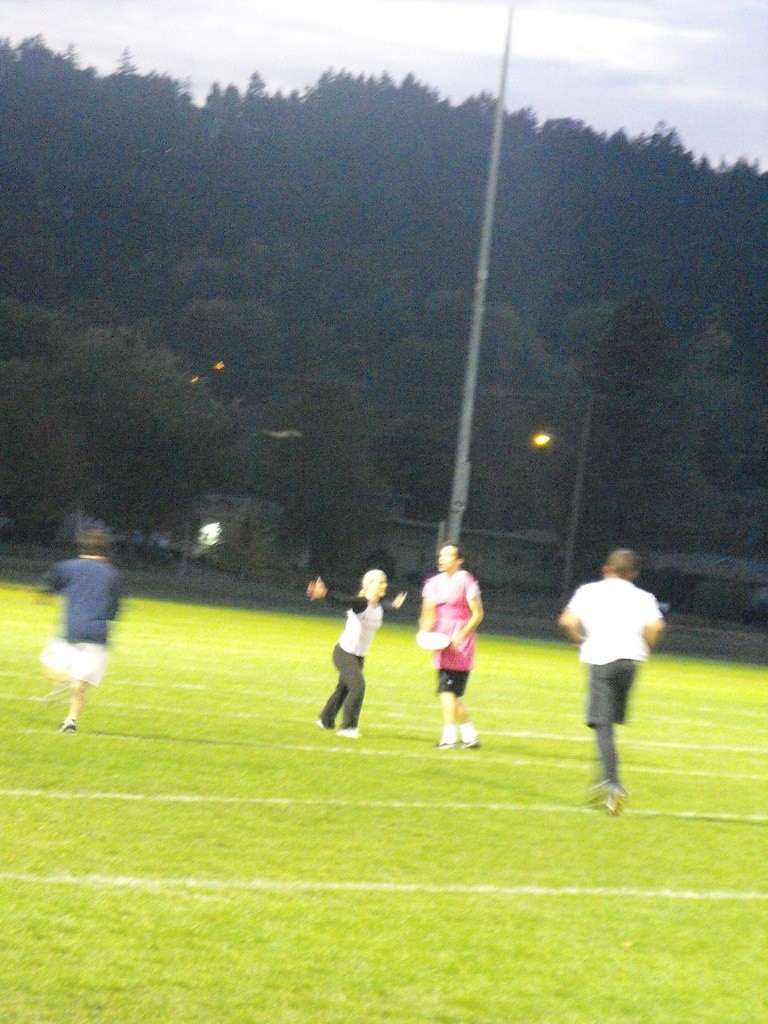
[317,719,324,729]
[604,781,626,811]
[337,727,359,737]
[434,740,459,750]
[59,717,76,733]
[460,738,480,749]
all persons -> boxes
[416,541,485,750]
[558,550,667,814]
[305,566,407,739]
[38,527,123,732]
